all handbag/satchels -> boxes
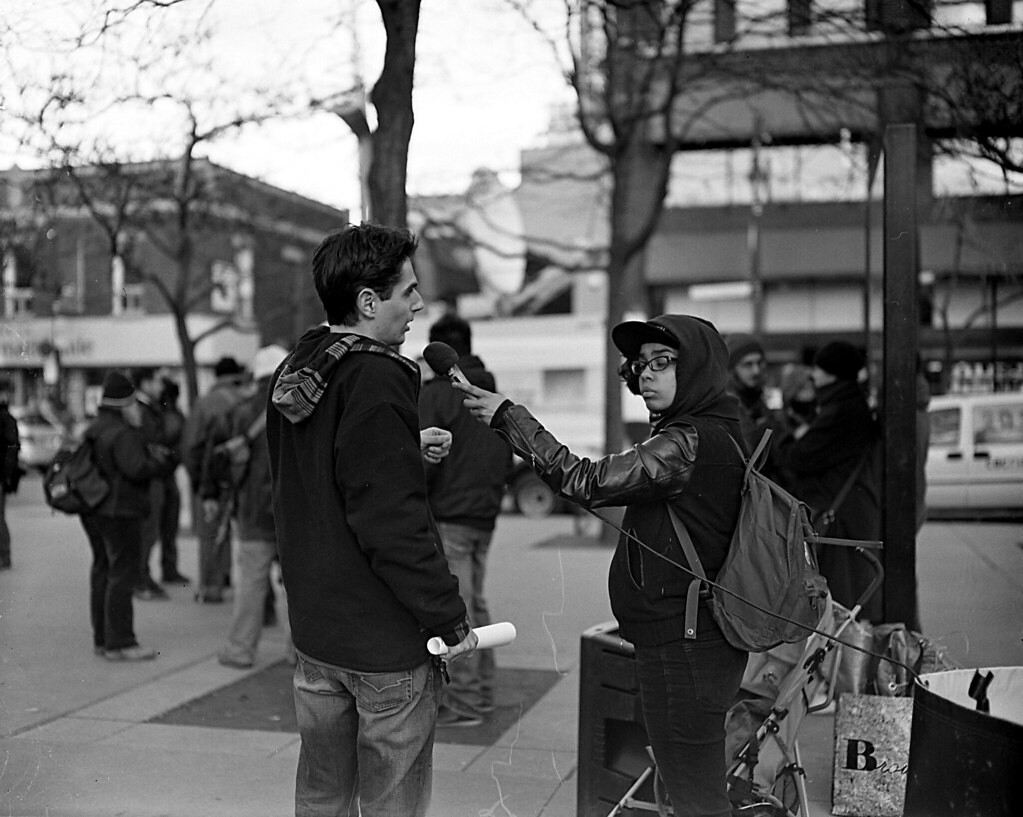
[869,623,924,697]
[832,692,914,817]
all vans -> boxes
[400,310,651,521]
[925,391,1022,520]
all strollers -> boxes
[603,544,885,817]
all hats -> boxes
[216,358,239,377]
[102,370,137,407]
[254,346,289,378]
[611,321,681,362]
[725,334,764,367]
[815,340,867,382]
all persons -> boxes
[0,378,23,567]
[266,224,479,817]
[419,315,515,727]
[453,315,750,817]
[728,332,931,622]
[183,344,299,667]
[79,369,191,660]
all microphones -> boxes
[424,341,480,401]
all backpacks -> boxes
[666,425,827,654]
[44,417,120,514]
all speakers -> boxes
[577,618,673,817]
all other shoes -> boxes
[136,578,164,598]
[163,572,186,584]
[94,644,105,654]
[435,705,483,727]
[219,652,252,669]
[474,702,495,711]
[194,590,223,603]
[105,644,156,660]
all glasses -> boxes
[630,356,680,376]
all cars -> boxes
[6,404,73,470]
